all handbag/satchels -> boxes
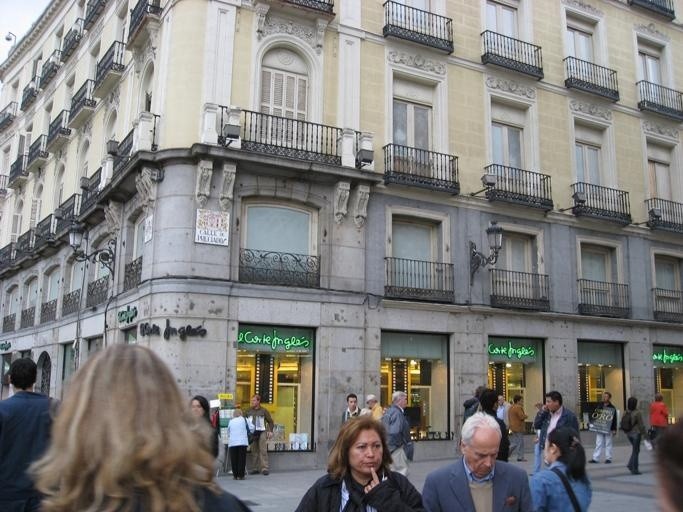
[647,425,656,439]
[244,417,255,444]
[531,435,539,444]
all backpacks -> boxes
[620,410,636,431]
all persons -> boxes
[529,428,592,512]
[1,358,59,511]
[383,390,411,477]
[462,387,580,476]
[227,409,255,480]
[653,418,683,512]
[297,416,423,512]
[646,394,669,451]
[341,394,382,425]
[8,341,250,511]
[422,412,532,512]
[588,393,617,463]
[623,398,647,476]
[186,395,219,460]
[243,395,273,475]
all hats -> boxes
[366,394,379,404]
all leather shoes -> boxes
[262,471,269,475]
[627,464,632,470]
[588,459,596,463]
[605,460,611,463]
[251,470,259,474]
[632,472,641,474]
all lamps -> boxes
[634,205,662,226]
[558,190,586,214]
[59,215,117,282]
[79,175,102,193]
[355,146,375,169]
[469,170,496,197]
[30,226,53,240]
[220,121,242,148]
[104,138,131,162]
[52,208,69,223]
[13,241,32,255]
[4,30,18,49]
[468,216,503,283]
